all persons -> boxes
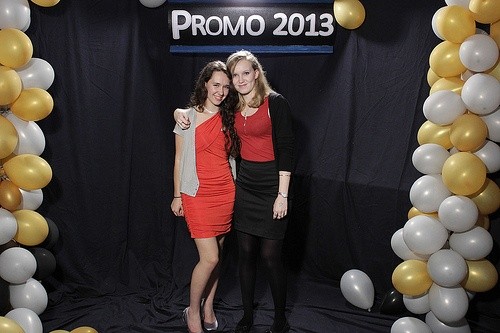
[173,50,296,333]
[171,60,241,333]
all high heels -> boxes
[184,307,204,333]
[201,298,218,331]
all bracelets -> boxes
[279,175,290,176]
[174,197,180,198]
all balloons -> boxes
[340,269,374,309]
[391,0,500,333]
[0,0,98,333]
[334,0,365,29]
[139,0,166,8]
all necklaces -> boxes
[203,106,218,113]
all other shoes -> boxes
[236,320,253,333]
[264,322,290,333]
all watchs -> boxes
[278,192,287,198]
